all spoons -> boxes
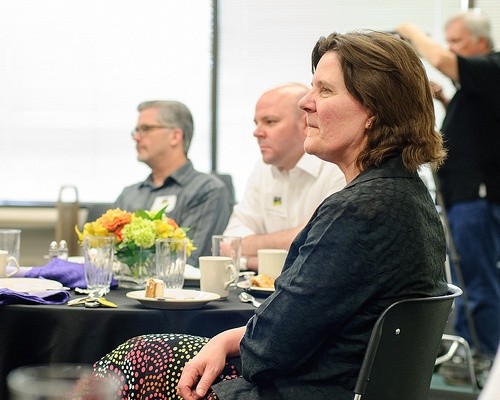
[239,291,261,308]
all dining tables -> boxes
[0,268,268,367]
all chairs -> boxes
[353,282,463,400]
[435,199,500,392]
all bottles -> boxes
[57,239,69,262]
[48,240,58,260]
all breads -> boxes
[251,274,275,289]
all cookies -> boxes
[145,277,165,299]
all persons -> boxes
[72,29,447,400]
[217,81,347,271]
[115,99,233,268]
[397,7,500,387]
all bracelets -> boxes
[240,256,249,272]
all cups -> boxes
[257,249,288,280]
[6,364,126,400]
[199,256,237,301]
[211,235,242,291]
[0,228,21,271]
[83,237,114,294]
[0,250,20,278]
[155,238,187,289]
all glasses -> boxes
[131,125,166,136]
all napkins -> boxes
[0,275,71,305]
[24,257,119,288]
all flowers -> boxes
[74,202,198,282]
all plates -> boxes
[236,281,275,294]
[126,288,220,309]
[183,275,200,282]
[0,278,63,294]
